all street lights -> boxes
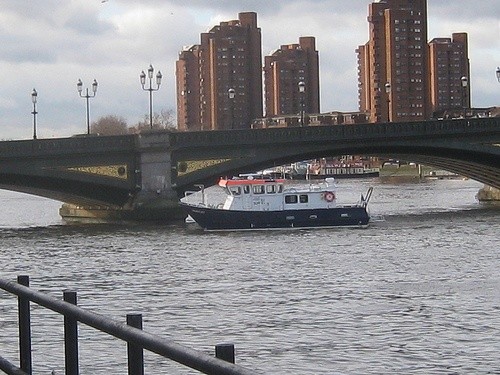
[385,81,392,122]
[139,64,162,132]
[30,88,38,139]
[461,74,468,119]
[227,87,236,129]
[298,81,306,126]
[77,79,98,135]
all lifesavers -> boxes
[324,192,335,202]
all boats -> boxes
[178,172,375,231]
[271,155,380,178]
